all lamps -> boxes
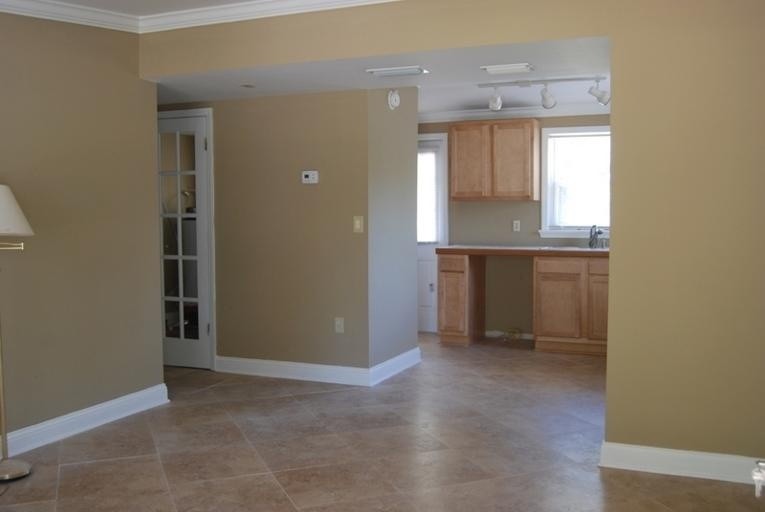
[478,76,611,112]
[0,183,35,484]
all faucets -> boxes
[589,226,603,248]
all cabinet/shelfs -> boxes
[533,255,609,355]
[448,118,540,202]
[437,253,485,345]
